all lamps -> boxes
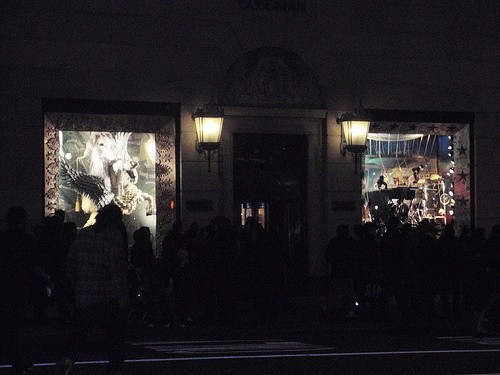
[335,103,373,174]
[191,98,226,172]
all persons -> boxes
[0,203,287,375]
[320,216,500,337]
